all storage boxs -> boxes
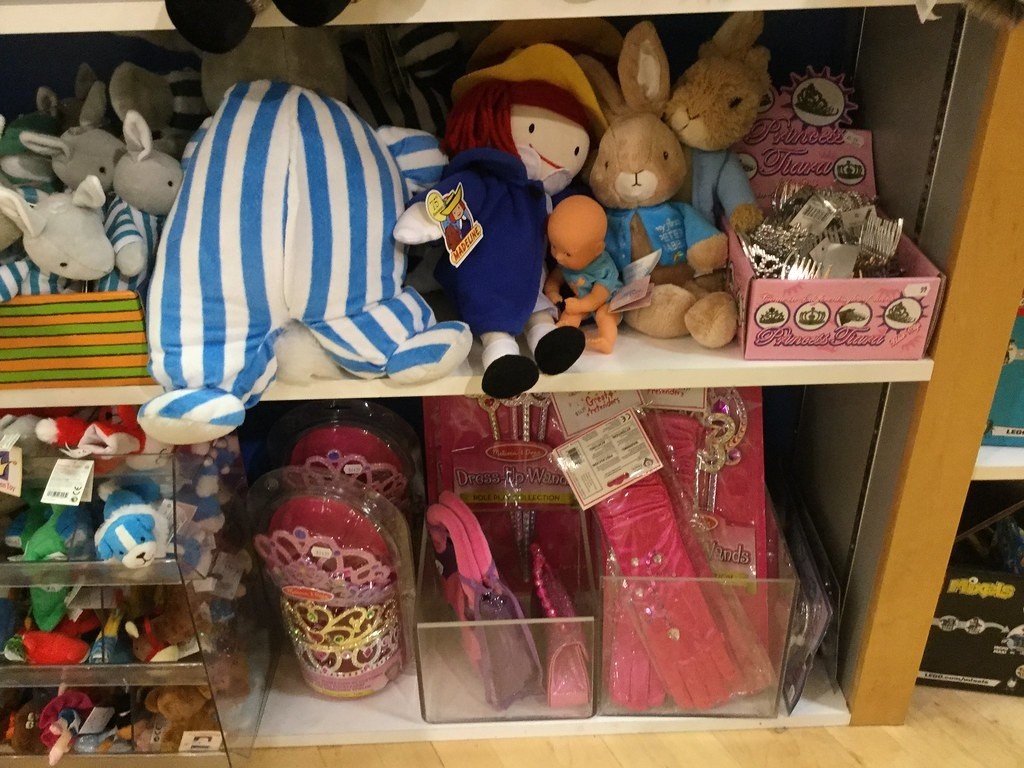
[914,483,1024,699]
[981,304,1024,448]
[720,66,947,361]
[0,425,280,768]
[414,491,598,722]
[605,490,799,721]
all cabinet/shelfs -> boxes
[0,0,1024,767]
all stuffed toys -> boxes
[165,0,356,54]
[138,23,472,444]
[0,33,210,306]
[0,406,270,764]
[392,10,771,400]
